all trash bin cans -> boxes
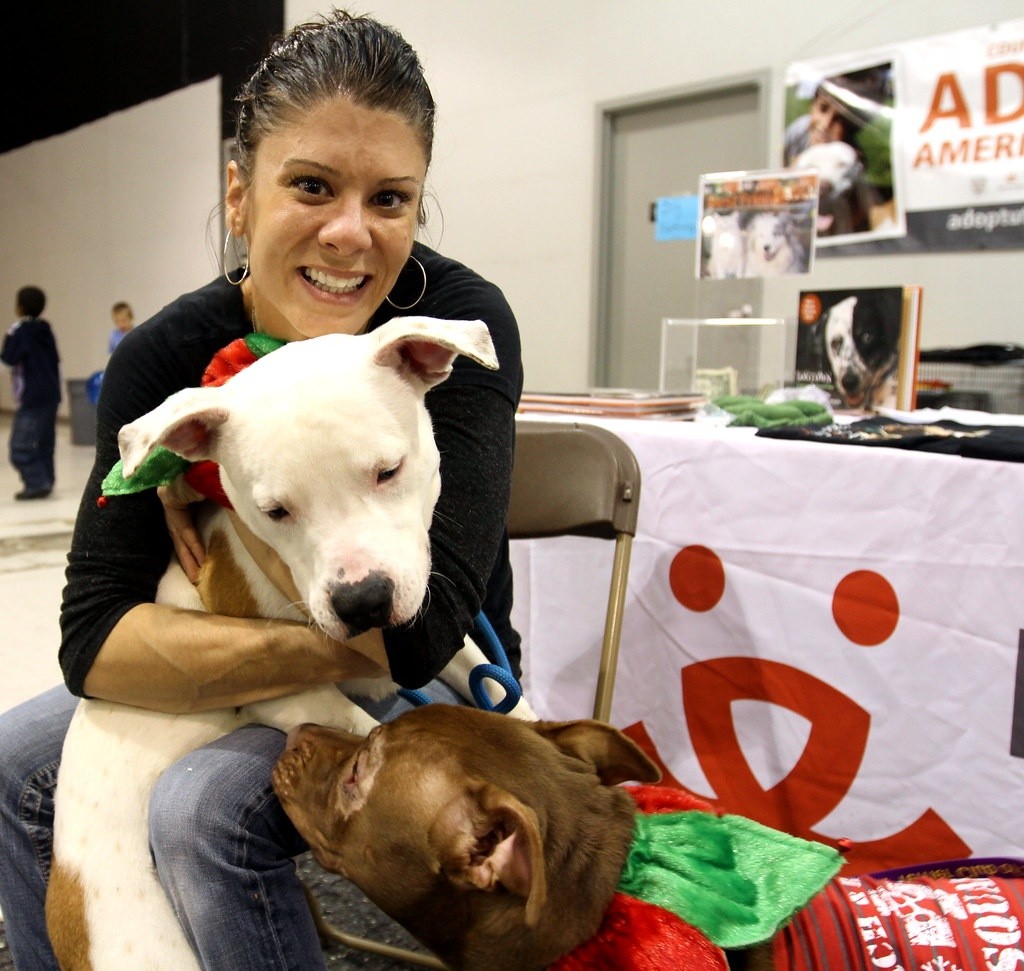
[65,380,98,446]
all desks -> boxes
[502,403,1024,848]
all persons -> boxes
[0,286,61,500]
[783,66,888,237]
[108,302,139,358]
[1,13,520,971]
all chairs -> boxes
[504,414,644,725]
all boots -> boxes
[43,456,55,484]
[16,460,51,500]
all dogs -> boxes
[43,316,501,971]
[271,702,1024,971]
[808,293,901,413]
[707,209,808,279]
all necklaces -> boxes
[250,296,257,333]
